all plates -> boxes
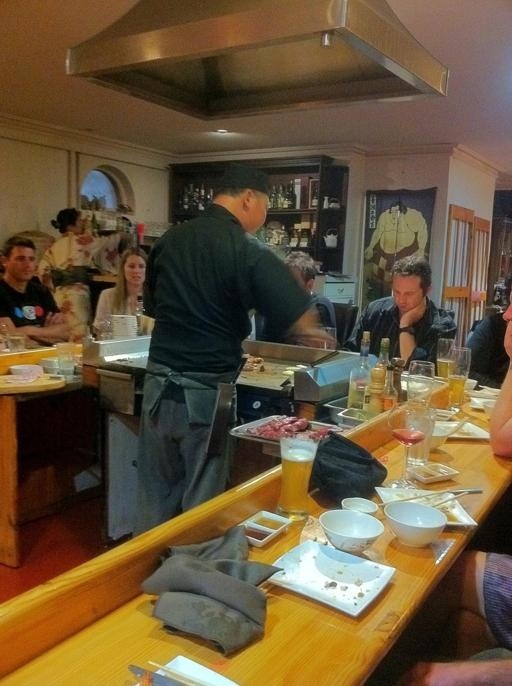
[232,482,479,619]
[111,315,139,342]
[414,380,500,485]
[132,652,244,686]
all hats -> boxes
[213,161,273,194]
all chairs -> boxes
[90,280,117,338]
[471,319,482,332]
[332,302,360,352]
[445,310,456,320]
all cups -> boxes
[388,338,472,486]
[277,436,319,523]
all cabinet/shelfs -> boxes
[160,151,349,274]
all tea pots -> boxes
[324,227,339,247]
[329,198,341,208]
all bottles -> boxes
[323,196,329,209]
[174,180,214,224]
[90,207,98,232]
[347,328,405,416]
[255,176,323,248]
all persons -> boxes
[91,247,149,339]
[0,236,70,349]
[256,251,338,349]
[131,163,327,540]
[36,207,134,341]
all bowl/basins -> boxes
[1,295,91,384]
